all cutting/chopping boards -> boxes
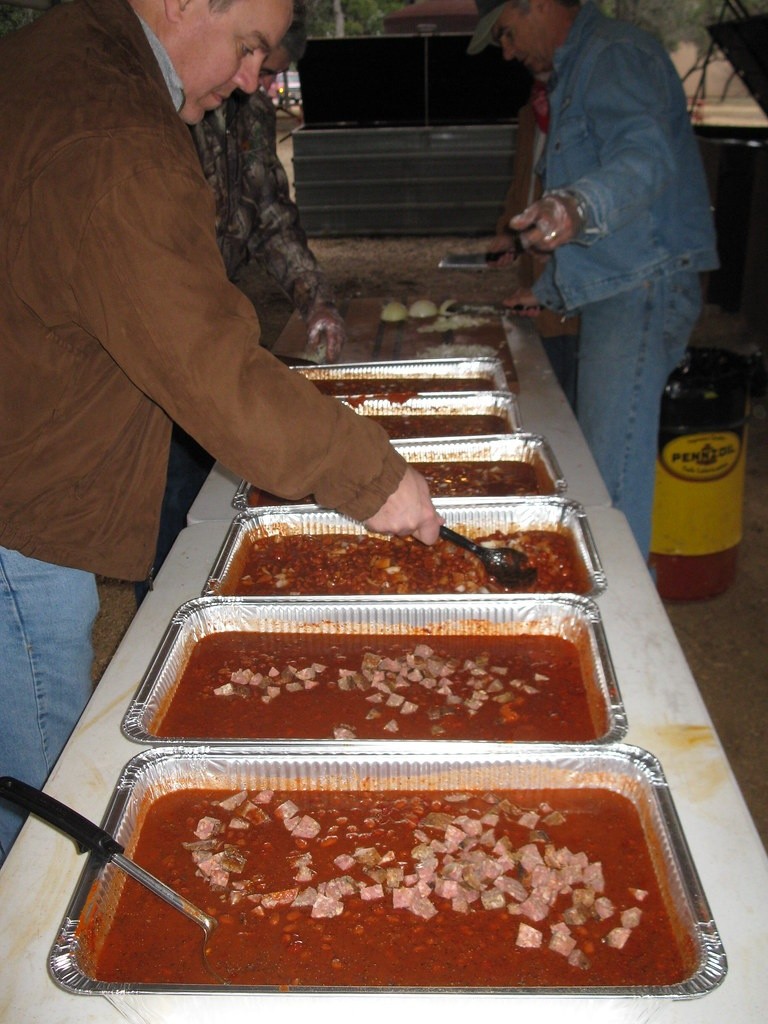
[270,298,518,395]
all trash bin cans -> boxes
[647,347,758,603]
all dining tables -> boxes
[186,295,613,525]
[0,506,768,1023]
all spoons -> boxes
[443,529,537,588]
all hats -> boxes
[466,0,509,57]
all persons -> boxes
[468,0,720,567]
[132,2,345,610]
[0,0,446,870]
[486,73,581,421]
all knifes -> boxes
[438,251,521,269]
[446,303,543,317]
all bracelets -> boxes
[542,186,590,223]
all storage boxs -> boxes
[291,35,518,236]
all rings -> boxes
[542,230,558,243]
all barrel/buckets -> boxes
[647,347,752,600]
